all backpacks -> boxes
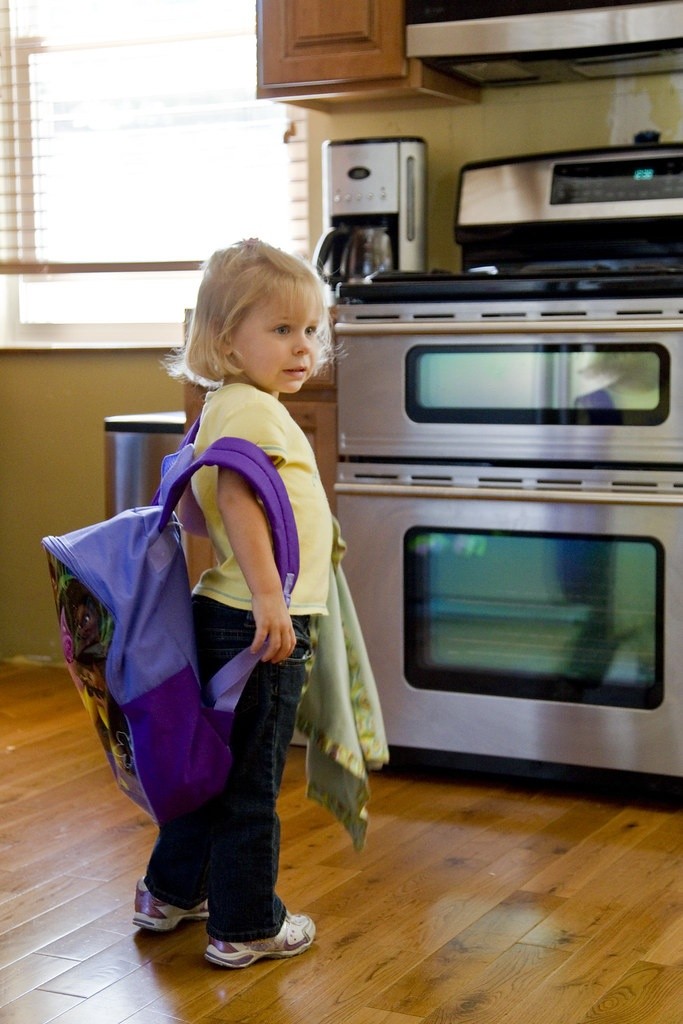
[43,417,298,825]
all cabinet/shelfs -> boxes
[255,0,482,115]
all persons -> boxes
[134,241,333,968]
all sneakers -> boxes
[133,877,209,932]
[205,909,316,968]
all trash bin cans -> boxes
[102,411,187,521]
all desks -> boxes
[103,411,186,519]
